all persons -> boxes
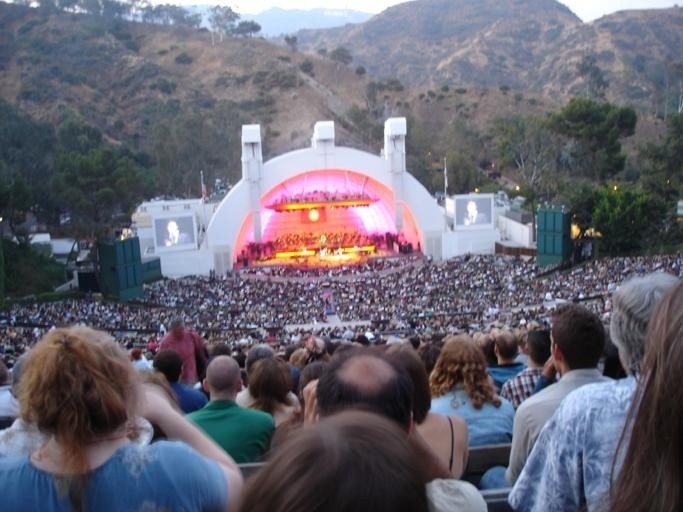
[461,199,488,225]
[1,275,683,511]
[0,232,682,416]
[162,220,190,245]
